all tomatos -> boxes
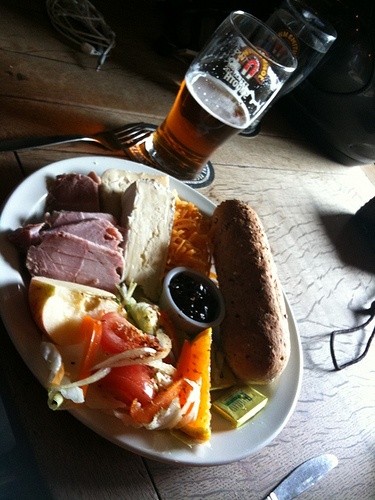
[89,313,157,406]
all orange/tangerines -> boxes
[171,328,214,443]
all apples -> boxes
[29,276,127,343]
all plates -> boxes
[0,156,303,466]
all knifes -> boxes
[264,454,338,500]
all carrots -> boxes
[163,199,217,280]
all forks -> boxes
[0,122,153,152]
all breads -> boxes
[209,199,290,384]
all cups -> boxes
[241,0,337,135]
[161,267,225,336]
[153,11,296,179]
